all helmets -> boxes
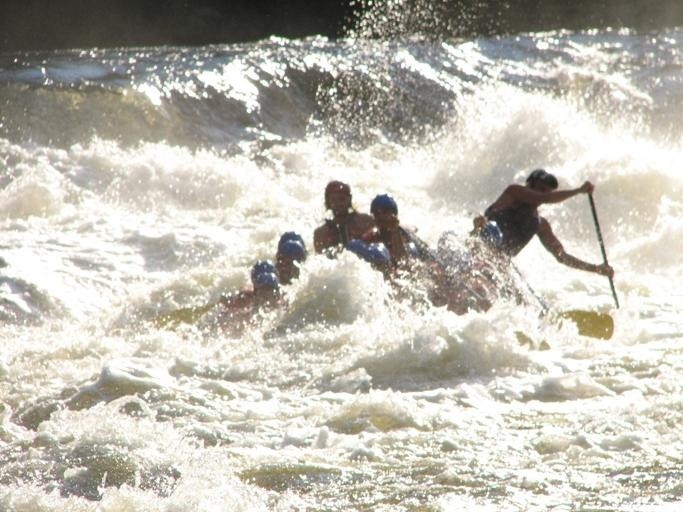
[279,233,306,259]
[526,170,558,188]
[346,240,390,265]
[252,259,279,290]
[371,194,397,215]
[325,181,350,206]
[481,221,504,247]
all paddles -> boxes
[158,295,226,328]
[543,310,615,342]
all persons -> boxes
[313,180,375,252]
[369,193,418,259]
[465,167,615,281]
[364,241,391,271]
[249,258,277,283]
[435,220,503,282]
[252,272,279,305]
[277,232,308,258]
[280,239,302,270]
[344,238,366,257]
